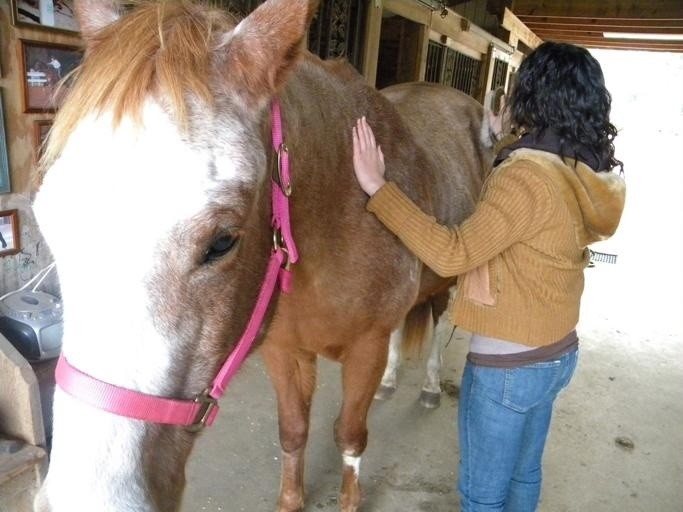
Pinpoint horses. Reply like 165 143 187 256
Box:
30 0 513 512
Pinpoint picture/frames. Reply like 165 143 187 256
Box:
8 0 133 39
0 208 21 257
33 119 55 160
0 85 12 196
16 38 85 113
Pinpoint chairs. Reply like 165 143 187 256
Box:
0 333 50 512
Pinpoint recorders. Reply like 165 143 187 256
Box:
0 288 65 362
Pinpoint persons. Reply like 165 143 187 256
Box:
46 57 62 79
350 39 625 512
